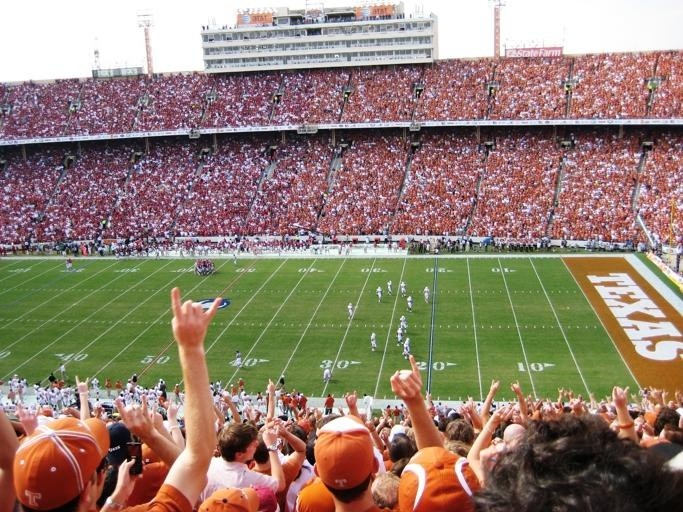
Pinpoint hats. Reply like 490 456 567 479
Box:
314 416 375 490
13 417 129 509
399 446 481 512
197 488 260 512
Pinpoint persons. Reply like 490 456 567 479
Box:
0 50 683 512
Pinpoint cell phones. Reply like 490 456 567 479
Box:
125 441 143 476
